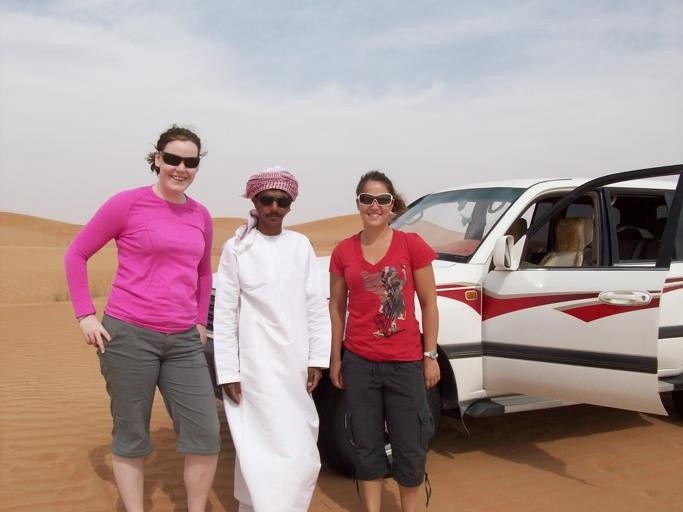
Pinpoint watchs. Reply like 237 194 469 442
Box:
424 351 439 360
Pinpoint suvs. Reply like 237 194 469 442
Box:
205 164 683 476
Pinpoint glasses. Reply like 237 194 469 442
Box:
256 195 291 208
159 151 200 168
356 193 393 207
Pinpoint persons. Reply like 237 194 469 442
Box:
64 124 221 512
213 167 332 512
329 171 441 511
